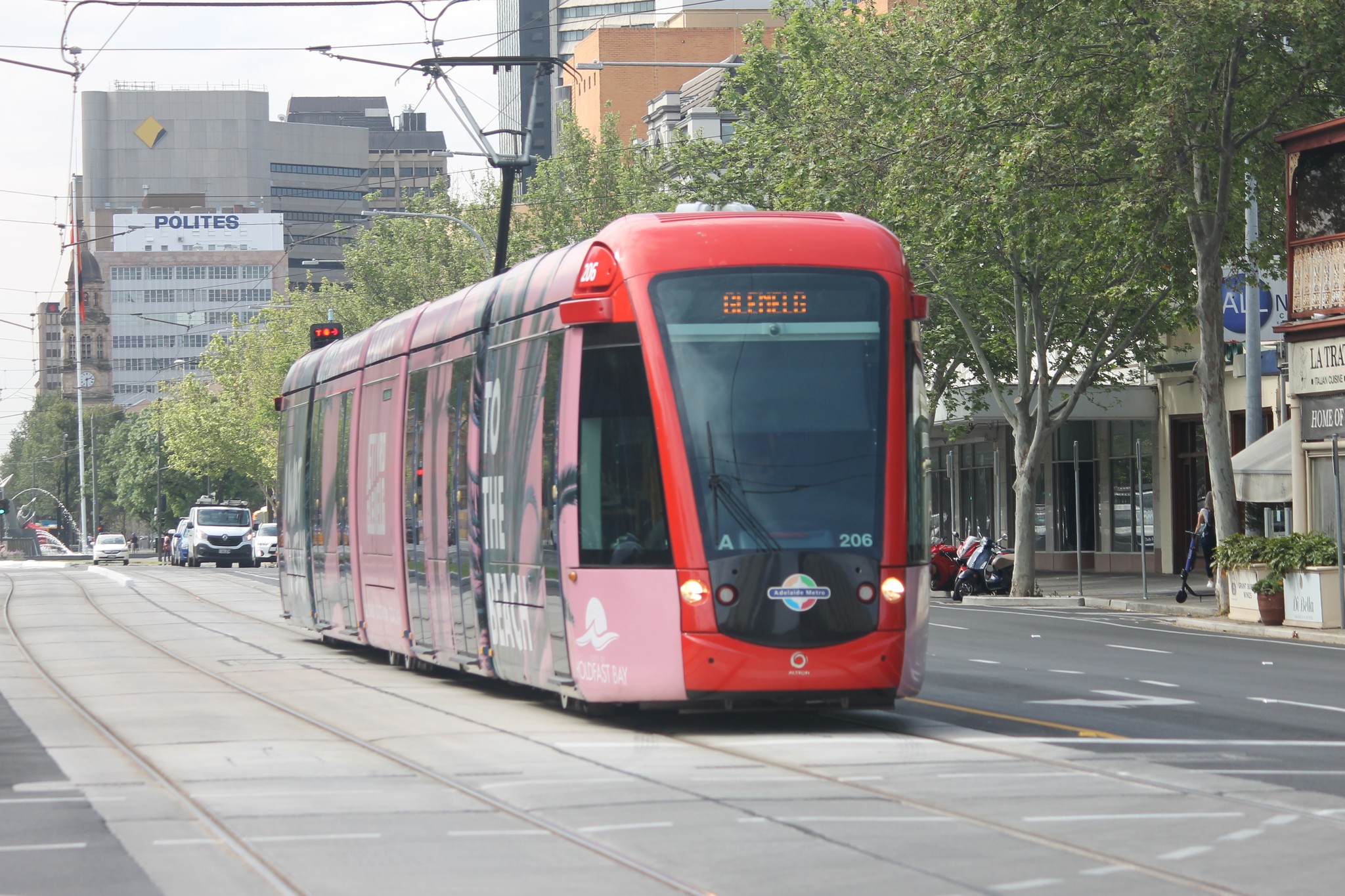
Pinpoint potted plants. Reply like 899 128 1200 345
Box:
1260 532 1345 630
1252 573 1285 626
1207 533 1274 623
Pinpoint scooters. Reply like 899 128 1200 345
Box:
952 531 1015 601
930 525 960 592
954 525 1015 602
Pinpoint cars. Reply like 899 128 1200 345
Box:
174 523 189 566
1035 504 1046 541
90 532 131 565
1099 504 1154 549
167 517 190 565
253 522 278 568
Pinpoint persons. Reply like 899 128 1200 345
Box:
130 533 138 553
163 532 172 563
1195 491 1216 588
87 533 93 548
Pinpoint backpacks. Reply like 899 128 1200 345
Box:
1205 508 1216 536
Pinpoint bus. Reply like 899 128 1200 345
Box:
271 56 929 733
251 506 277 528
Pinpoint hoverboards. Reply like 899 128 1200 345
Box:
1175 530 1215 603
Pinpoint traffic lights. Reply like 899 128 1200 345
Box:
4 498 10 514
0 498 5 514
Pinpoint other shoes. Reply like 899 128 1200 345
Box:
1207 580 1215 588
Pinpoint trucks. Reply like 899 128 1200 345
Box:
187 492 253 568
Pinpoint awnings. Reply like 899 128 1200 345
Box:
1226 416 1292 502
